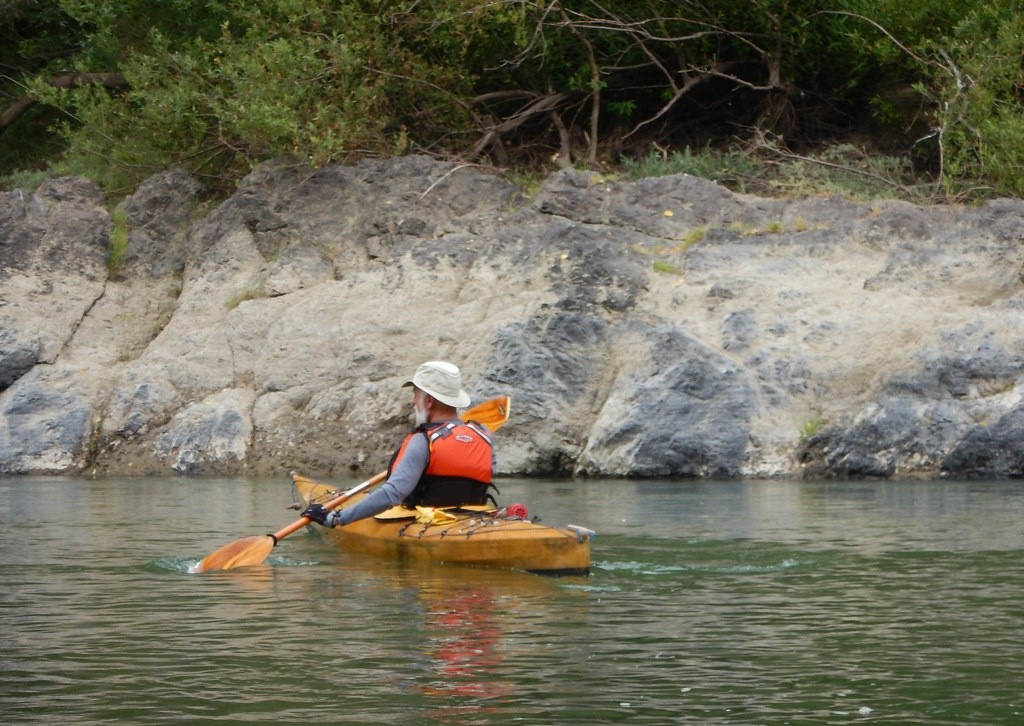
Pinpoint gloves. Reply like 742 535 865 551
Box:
300 502 336 529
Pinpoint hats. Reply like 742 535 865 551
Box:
402 360 472 409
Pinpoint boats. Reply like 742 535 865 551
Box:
286 469 594 578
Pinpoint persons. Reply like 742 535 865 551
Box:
301 361 497 530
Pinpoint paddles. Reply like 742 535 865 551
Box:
189 393 512 573
